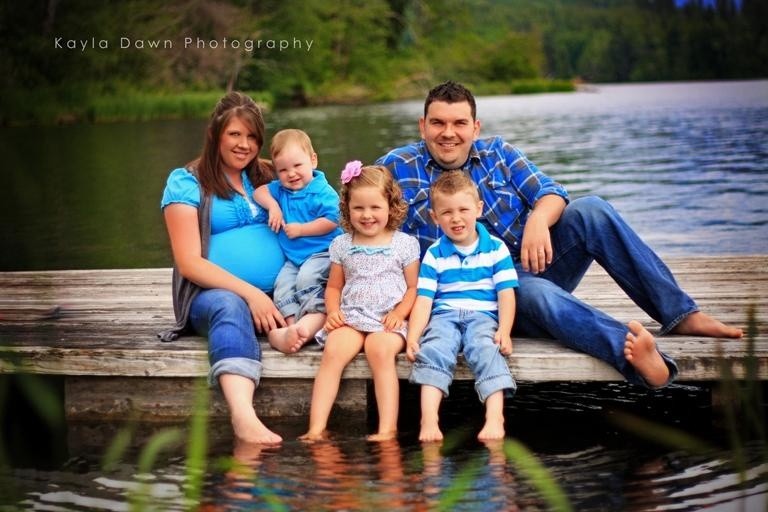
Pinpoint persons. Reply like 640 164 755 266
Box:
299 165 421 441
156 93 327 445
252 128 345 326
405 171 520 441
373 82 744 389
